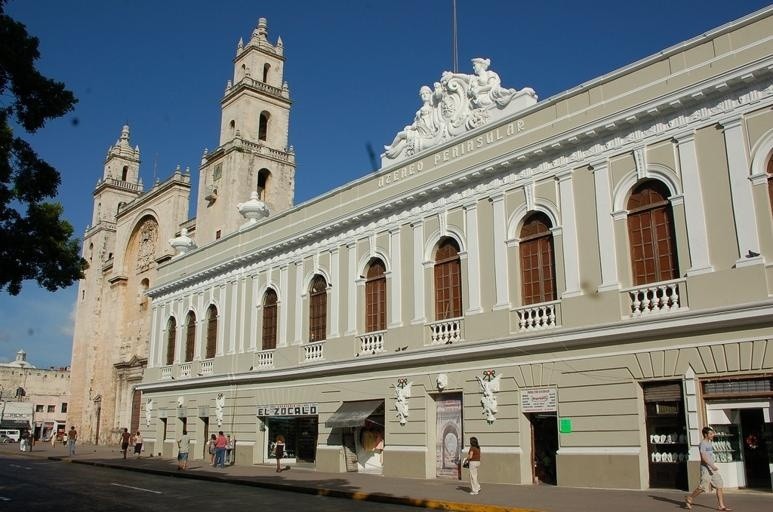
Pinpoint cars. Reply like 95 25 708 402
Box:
0 435 11 444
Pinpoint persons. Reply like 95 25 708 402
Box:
383 85 444 159
206 434 217 466
441 58 515 108
684 427 732 511
43 426 78 456
464 437 481 495
20 429 30 452
119 428 131 460
213 430 228 469
176 430 190 471
271 435 287 473
132 431 142 458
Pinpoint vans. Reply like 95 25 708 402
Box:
0 429 20 443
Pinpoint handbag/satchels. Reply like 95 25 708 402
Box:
463 459 469 468
